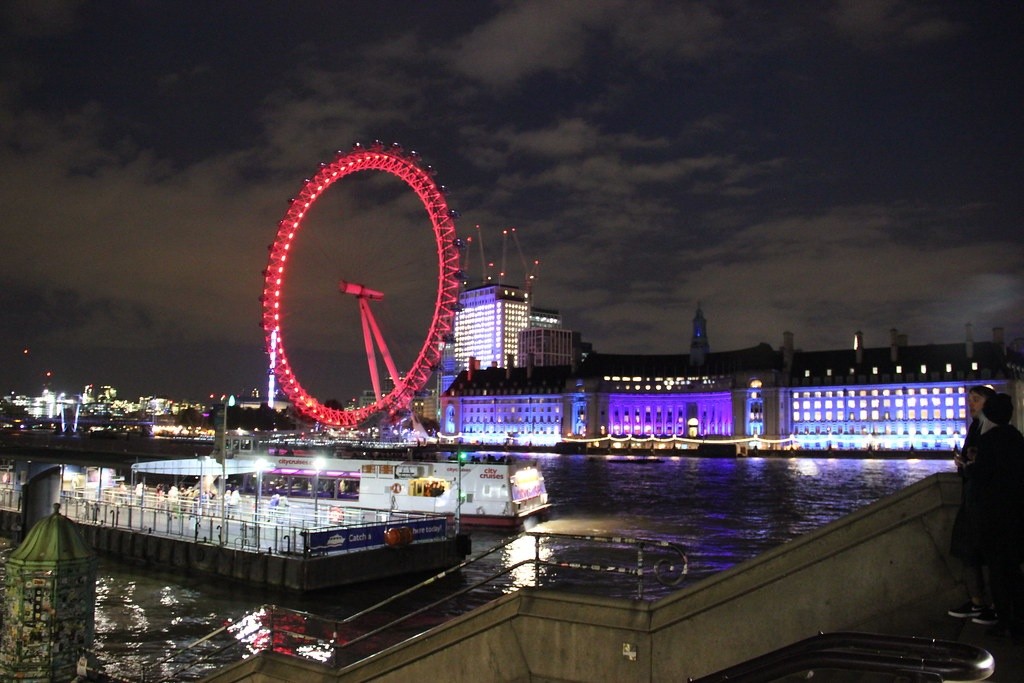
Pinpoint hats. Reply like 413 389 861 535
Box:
981 393 1015 426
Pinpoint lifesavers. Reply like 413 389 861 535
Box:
398 526 413 544
391 482 402 495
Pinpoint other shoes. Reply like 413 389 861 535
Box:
947 595 991 620
971 606 1000 627
984 623 1013 646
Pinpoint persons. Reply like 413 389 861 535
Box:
224 490 241 518
136 482 146 505
471 454 512 465
156 483 213 512
267 494 289 523
119 482 128 503
275 476 360 493
423 482 444 497
